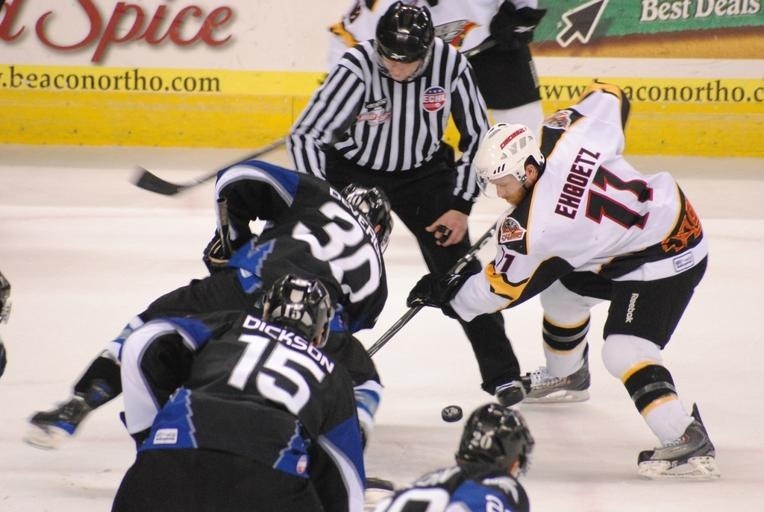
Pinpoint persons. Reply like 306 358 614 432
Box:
28 160 395 437
114 276 370 512
375 402 533 510
328 1 552 152
404 82 721 464
288 2 526 407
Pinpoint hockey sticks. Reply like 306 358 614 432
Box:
129 37 495 196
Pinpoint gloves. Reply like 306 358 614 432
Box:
490 1 547 61
406 271 471 319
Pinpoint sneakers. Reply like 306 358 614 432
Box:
521 346 590 399
495 381 526 407
30 399 89 432
637 402 715 464
365 477 393 490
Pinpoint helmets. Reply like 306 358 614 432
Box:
454 403 535 480
340 182 395 254
374 1 435 83
260 274 333 349
473 122 545 199
